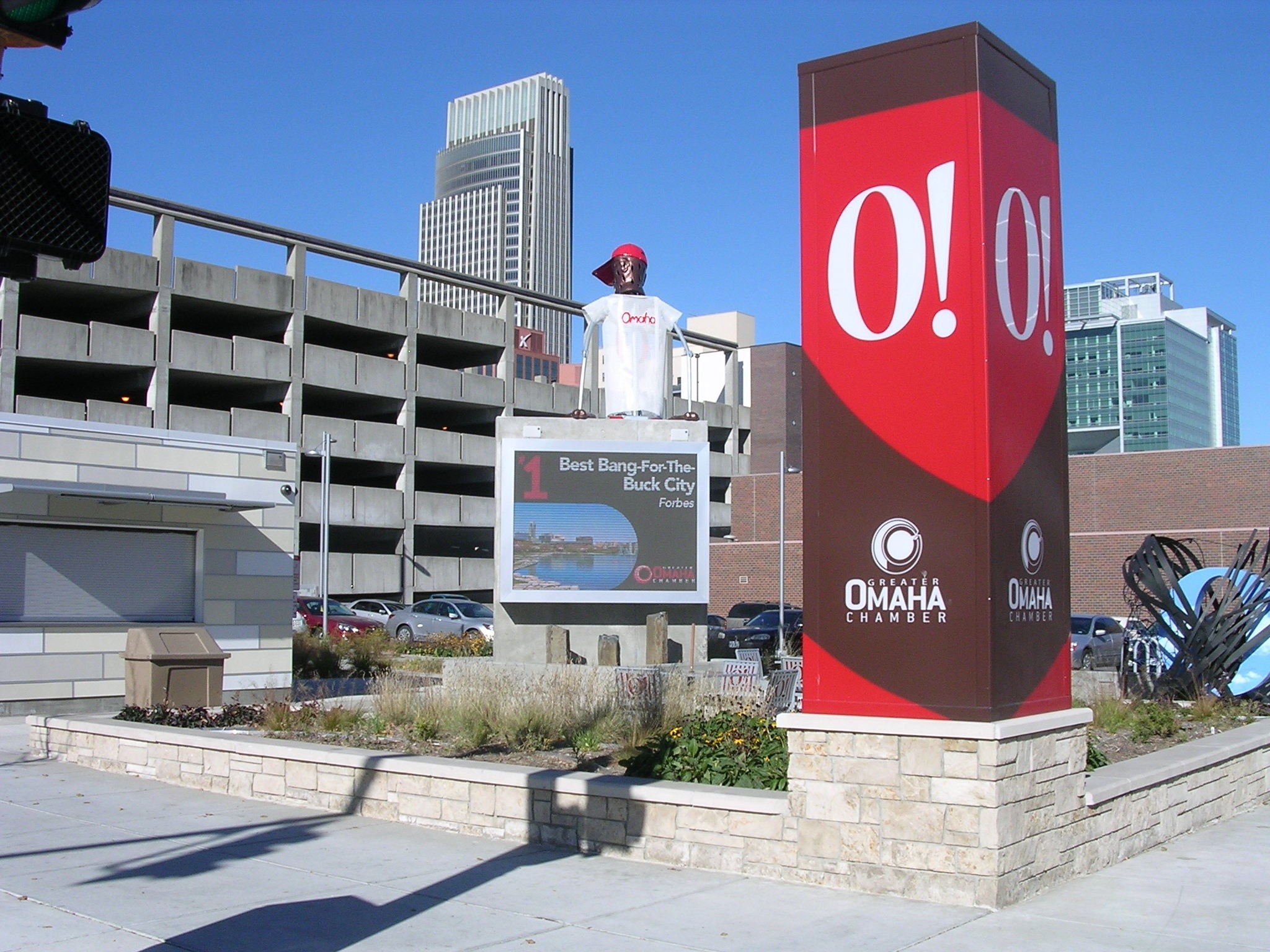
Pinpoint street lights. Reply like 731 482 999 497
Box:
777 451 800 653
305 433 337 642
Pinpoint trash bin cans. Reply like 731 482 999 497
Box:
119 627 231 709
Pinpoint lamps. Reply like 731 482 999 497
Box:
442 426 448 430
121 397 130 402
387 352 394 358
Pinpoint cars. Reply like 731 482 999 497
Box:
1111 616 1150 643
384 599 494 646
343 598 408 626
295 596 391 649
708 614 727 657
1070 613 1132 671
715 608 806 663
428 593 470 603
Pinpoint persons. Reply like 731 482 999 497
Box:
572 244 699 421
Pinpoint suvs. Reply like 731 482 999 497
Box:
724 600 803 631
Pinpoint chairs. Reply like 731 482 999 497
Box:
737 668 802 720
611 667 679 727
311 604 320 615
438 605 449 617
734 646 768 683
463 607 475 616
779 656 803 710
700 657 762 717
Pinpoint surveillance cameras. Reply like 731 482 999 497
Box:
281 485 291 495
